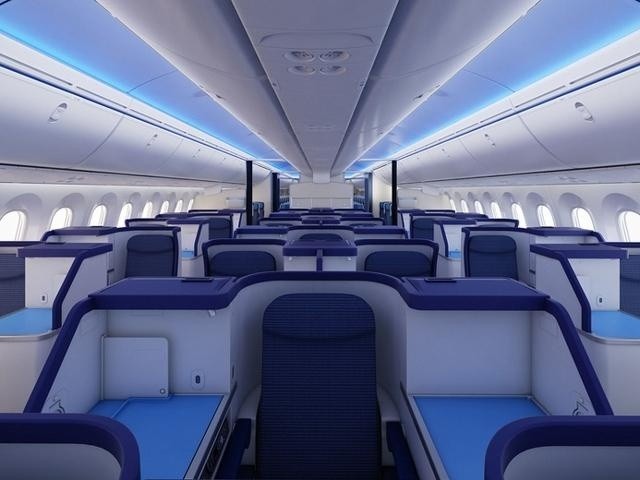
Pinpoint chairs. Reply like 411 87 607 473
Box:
234 225 407 242
462 228 606 286
0 276 238 479
0 238 114 337
252 202 265 225
410 213 489 242
380 201 417 228
271 211 372 217
380 277 640 480
229 270 398 480
259 217 385 226
278 209 365 212
397 209 455 237
188 209 247 232
155 213 233 238
201 240 286 278
355 237 440 281
530 242 640 338
432 219 519 277
125 219 209 277
41 226 181 285
